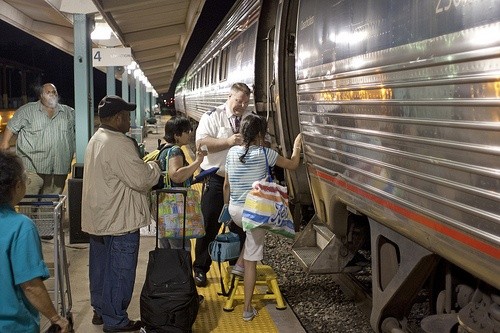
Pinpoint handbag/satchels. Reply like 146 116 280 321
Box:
210 224 240 263
151 187 206 239
242 177 295 243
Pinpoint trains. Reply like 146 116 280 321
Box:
173 0 500 291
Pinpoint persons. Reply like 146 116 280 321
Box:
193 83 260 288
0 83 75 240
223 113 301 321
150 116 208 307
0 149 69 333
81 96 161 333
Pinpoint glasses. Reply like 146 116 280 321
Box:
21 177 31 185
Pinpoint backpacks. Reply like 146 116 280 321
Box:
142 144 172 221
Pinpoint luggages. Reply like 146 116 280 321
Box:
140 189 199 333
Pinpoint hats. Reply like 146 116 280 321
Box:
98 95 136 118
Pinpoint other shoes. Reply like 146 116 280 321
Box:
242 305 258 322
103 319 142 333
93 309 104 325
232 264 245 277
194 268 208 287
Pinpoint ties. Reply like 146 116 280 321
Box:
235 116 240 133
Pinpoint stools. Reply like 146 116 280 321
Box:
218 264 287 313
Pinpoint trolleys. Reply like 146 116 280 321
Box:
15 194 74 333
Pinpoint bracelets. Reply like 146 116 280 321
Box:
51 316 61 323
293 147 301 150
